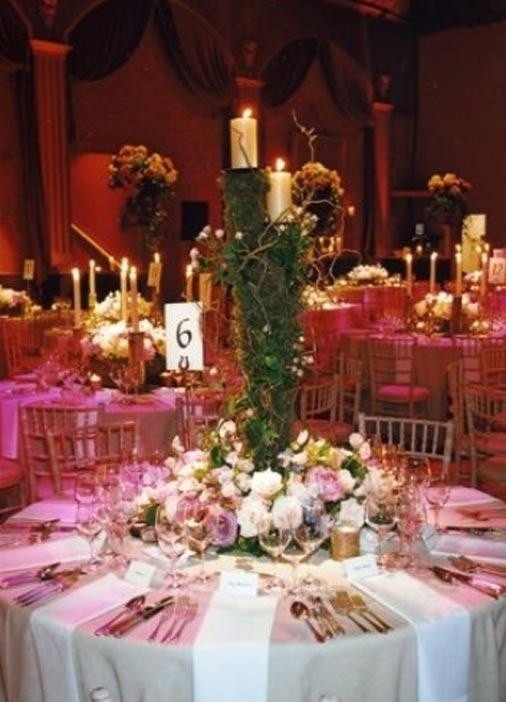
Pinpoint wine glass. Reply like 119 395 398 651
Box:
73 446 218 595
32 346 146 408
256 432 451 595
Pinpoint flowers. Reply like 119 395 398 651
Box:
107 143 180 266
427 172 472 212
76 416 409 558
293 161 345 236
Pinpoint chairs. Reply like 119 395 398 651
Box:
0 287 506 505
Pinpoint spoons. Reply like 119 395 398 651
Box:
94 595 146 635
290 601 324 644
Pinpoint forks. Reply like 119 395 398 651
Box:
329 588 395 636
149 596 199 646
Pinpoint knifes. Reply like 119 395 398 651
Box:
14 569 79 607
307 595 343 639
107 592 175 636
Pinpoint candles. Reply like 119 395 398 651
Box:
404 241 492 334
72 247 208 326
225 106 294 234
